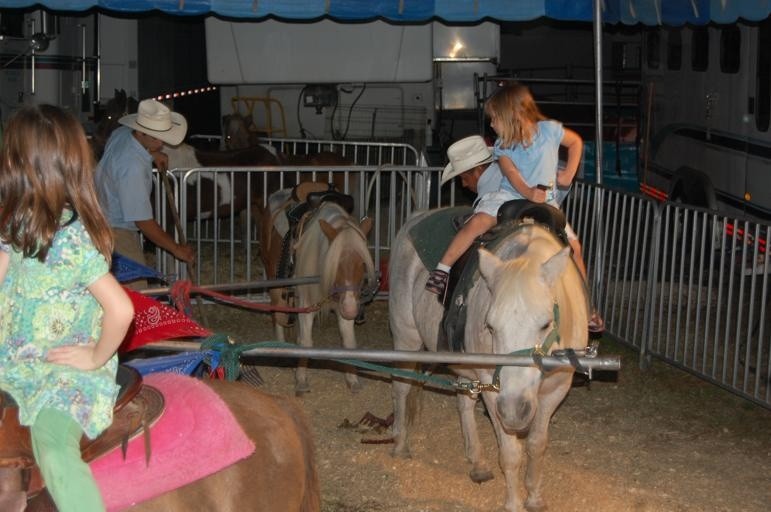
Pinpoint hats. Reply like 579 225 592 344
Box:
441 135 494 187
117 99 188 146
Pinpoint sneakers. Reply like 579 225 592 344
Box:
588 307 605 333
425 269 448 295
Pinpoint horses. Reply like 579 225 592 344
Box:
387 207 592 512
92 89 292 227
259 187 375 398
221 112 358 256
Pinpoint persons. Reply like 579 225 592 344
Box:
424 79 605 333
91 98 194 295
438 135 496 194
0 101 135 512
